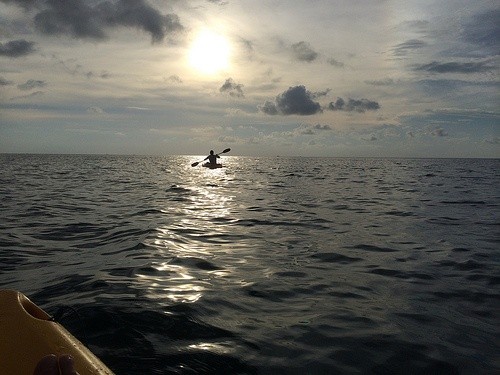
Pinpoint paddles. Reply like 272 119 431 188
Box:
191 148 231 167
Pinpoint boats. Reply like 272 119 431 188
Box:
202 162 222 168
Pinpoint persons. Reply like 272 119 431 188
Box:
204 150 220 164
